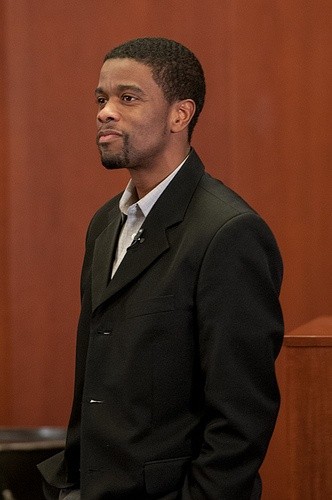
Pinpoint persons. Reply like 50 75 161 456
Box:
36 39 285 500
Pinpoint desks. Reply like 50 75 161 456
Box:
259 316 332 500
